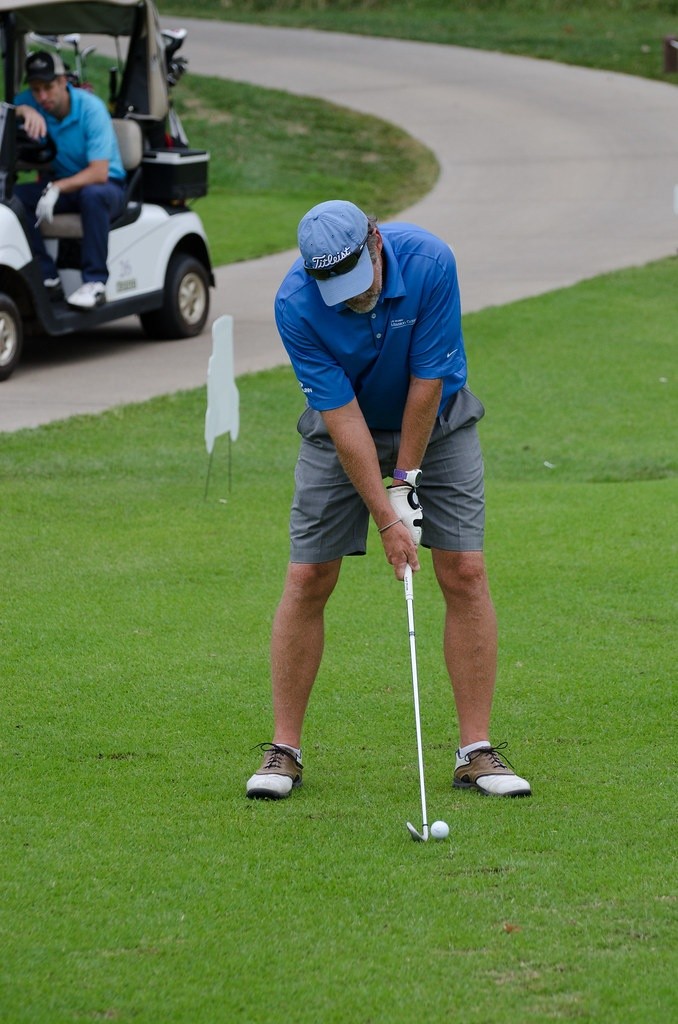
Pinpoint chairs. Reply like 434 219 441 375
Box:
35 117 144 239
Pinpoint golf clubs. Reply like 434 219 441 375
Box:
404 565 429 842
64 30 96 86
166 55 188 88
430 821 449 838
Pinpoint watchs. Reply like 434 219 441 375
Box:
393 468 422 487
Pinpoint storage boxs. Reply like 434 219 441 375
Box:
141 147 211 201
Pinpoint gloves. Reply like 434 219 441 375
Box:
35 186 60 225
386 484 423 547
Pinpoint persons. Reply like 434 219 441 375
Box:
244 201 530 800
15 49 128 310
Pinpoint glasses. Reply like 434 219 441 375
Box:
302 227 375 279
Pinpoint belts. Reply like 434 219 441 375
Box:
436 392 458 426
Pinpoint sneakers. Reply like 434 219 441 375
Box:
246 742 303 799
67 279 105 308
452 742 532 798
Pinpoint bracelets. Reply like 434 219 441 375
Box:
378 519 401 532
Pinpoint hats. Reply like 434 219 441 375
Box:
24 50 65 84
297 200 376 307
